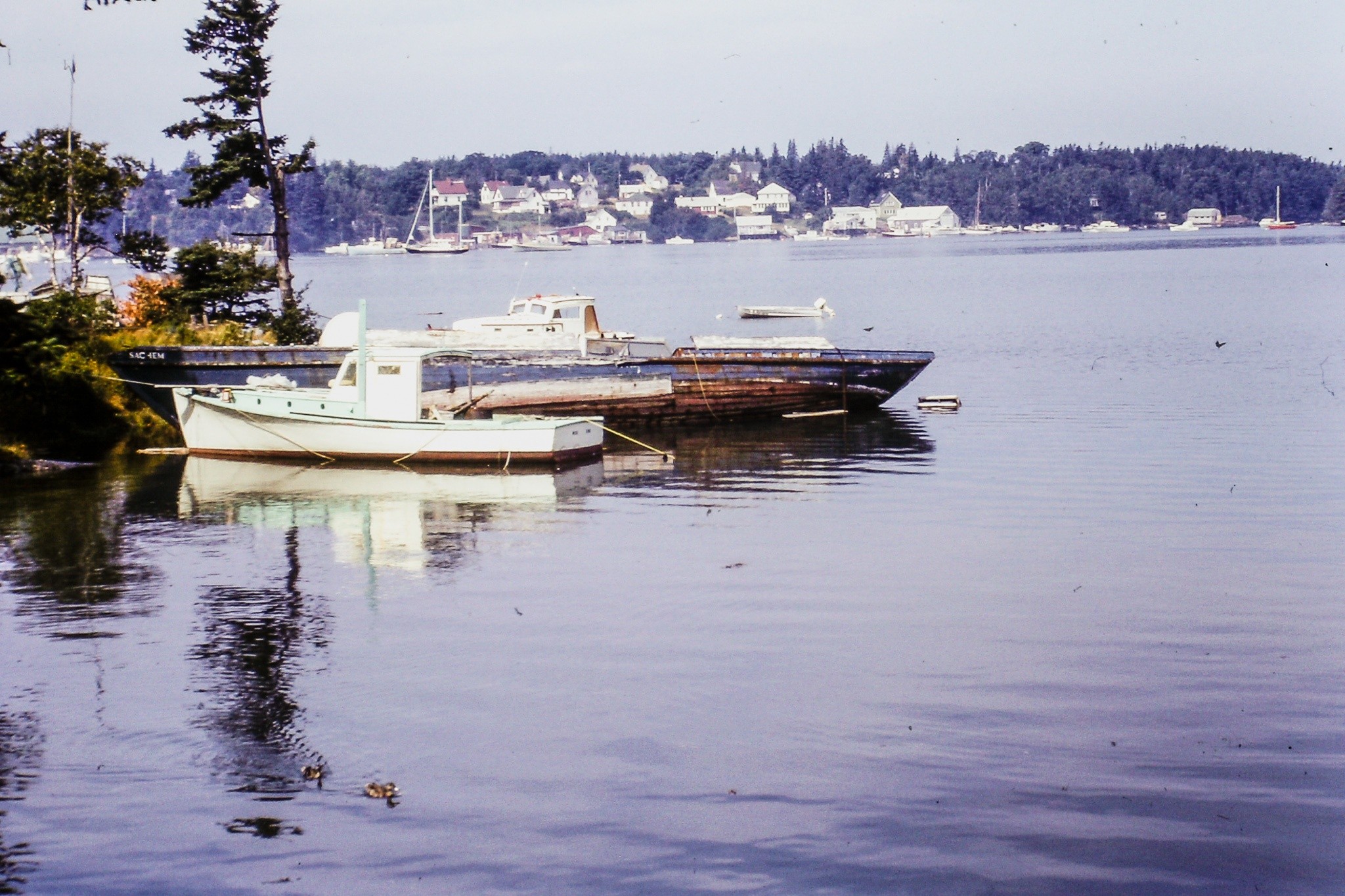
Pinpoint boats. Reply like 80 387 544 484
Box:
1170 221 1199 232
736 297 835 318
1024 222 1061 232
665 236 694 245
491 237 519 248
451 293 633 355
511 235 572 253
1259 218 1275 227
1080 221 1130 233
794 230 850 240
562 234 611 245
108 311 934 432
324 237 407 256
882 226 1017 236
173 298 605 468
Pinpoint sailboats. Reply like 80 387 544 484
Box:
961 181 997 234
402 169 470 254
1268 186 1297 229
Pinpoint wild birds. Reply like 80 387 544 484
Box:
1215 340 1227 349
863 327 874 332
515 608 522 616
706 507 721 517
1091 356 1108 370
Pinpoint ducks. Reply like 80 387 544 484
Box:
301 764 324 780
386 792 402 808
364 782 400 798
